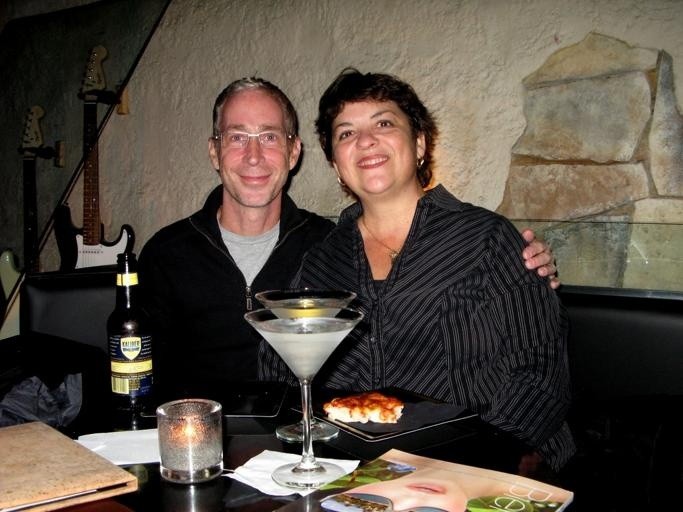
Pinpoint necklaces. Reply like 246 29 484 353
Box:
362 216 400 265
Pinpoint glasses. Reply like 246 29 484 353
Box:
212 131 293 147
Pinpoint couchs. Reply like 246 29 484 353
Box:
16 257 682 512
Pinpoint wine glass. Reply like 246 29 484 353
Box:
249 286 360 442
242 305 364 495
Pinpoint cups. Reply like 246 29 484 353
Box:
157 399 229 485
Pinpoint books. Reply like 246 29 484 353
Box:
271 447 575 512
0 422 139 511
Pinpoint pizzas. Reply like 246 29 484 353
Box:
323 390 405 424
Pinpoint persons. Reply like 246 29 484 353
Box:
134 75 561 386
320 448 573 512
511 451 541 476
250 67 578 478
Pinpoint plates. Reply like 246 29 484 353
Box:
288 386 481 445
139 379 290 418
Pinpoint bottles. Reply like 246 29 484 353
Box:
112 412 154 498
105 254 153 413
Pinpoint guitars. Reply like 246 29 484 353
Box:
1 107 51 339
53 46 135 268
